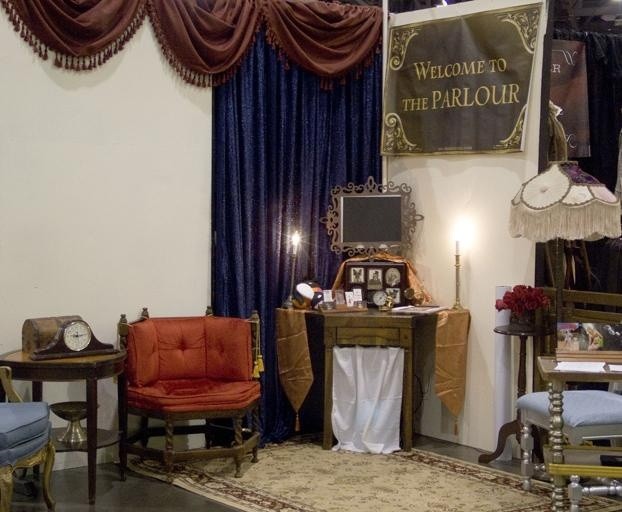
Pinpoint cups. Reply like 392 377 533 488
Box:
335 290 344 305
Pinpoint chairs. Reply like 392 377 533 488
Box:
0 367 55 511
113 303 263 485
475 376 622 512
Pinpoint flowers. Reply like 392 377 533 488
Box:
491 282 552 312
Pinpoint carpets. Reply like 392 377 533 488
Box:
113 447 622 511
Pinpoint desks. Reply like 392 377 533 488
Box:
533 353 622 511
0 348 129 505
479 325 554 465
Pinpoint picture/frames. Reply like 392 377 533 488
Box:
349 267 383 292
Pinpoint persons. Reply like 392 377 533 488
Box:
369 271 381 285
587 328 603 350
561 331 574 348
606 325 620 341
